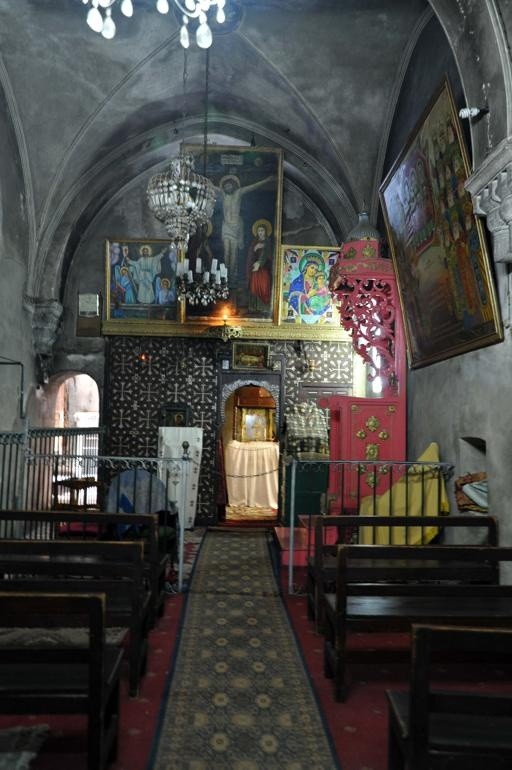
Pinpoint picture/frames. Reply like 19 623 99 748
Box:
178 142 284 332
104 233 184 325
376 69 505 369
277 245 347 329
231 341 271 373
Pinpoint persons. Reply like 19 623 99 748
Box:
175 415 186 426
111 173 338 323
395 117 494 331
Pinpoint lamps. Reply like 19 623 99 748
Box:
150 26 217 243
75 1 226 54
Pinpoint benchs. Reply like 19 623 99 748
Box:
307 513 511 770
0 508 178 768
